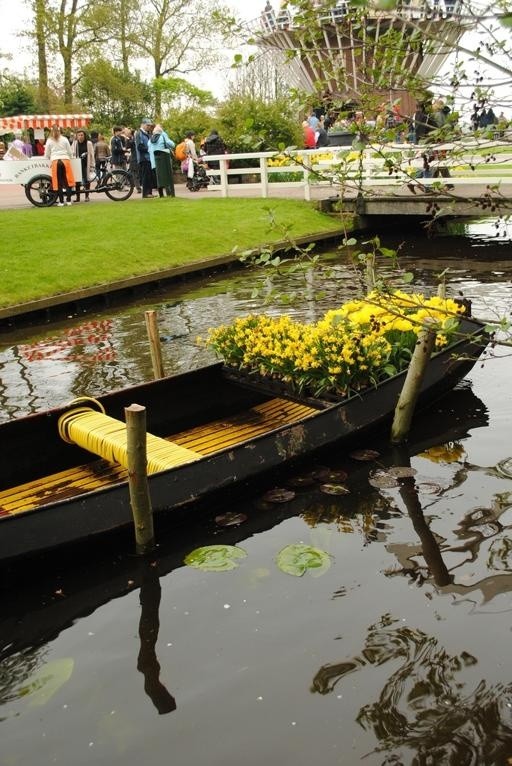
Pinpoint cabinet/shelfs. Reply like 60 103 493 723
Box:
0 155 91 186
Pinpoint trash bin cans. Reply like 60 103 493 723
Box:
154 150 175 198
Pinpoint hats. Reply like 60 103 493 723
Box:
142 118 152 126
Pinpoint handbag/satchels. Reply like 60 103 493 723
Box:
88 171 99 182
181 158 189 171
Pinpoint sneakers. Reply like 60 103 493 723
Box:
407 184 455 194
57 202 71 206
122 185 130 191
137 188 158 198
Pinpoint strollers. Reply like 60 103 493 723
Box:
185 154 215 192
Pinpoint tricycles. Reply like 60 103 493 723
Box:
0 113 136 207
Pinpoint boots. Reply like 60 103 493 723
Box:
84 183 90 202
73 185 80 202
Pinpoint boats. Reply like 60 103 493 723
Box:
2 304 493 564
0 379 486 665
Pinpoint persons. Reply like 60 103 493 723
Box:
1 117 231 207
299 100 506 149
263 0 276 25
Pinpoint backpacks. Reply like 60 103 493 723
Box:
176 139 191 161
